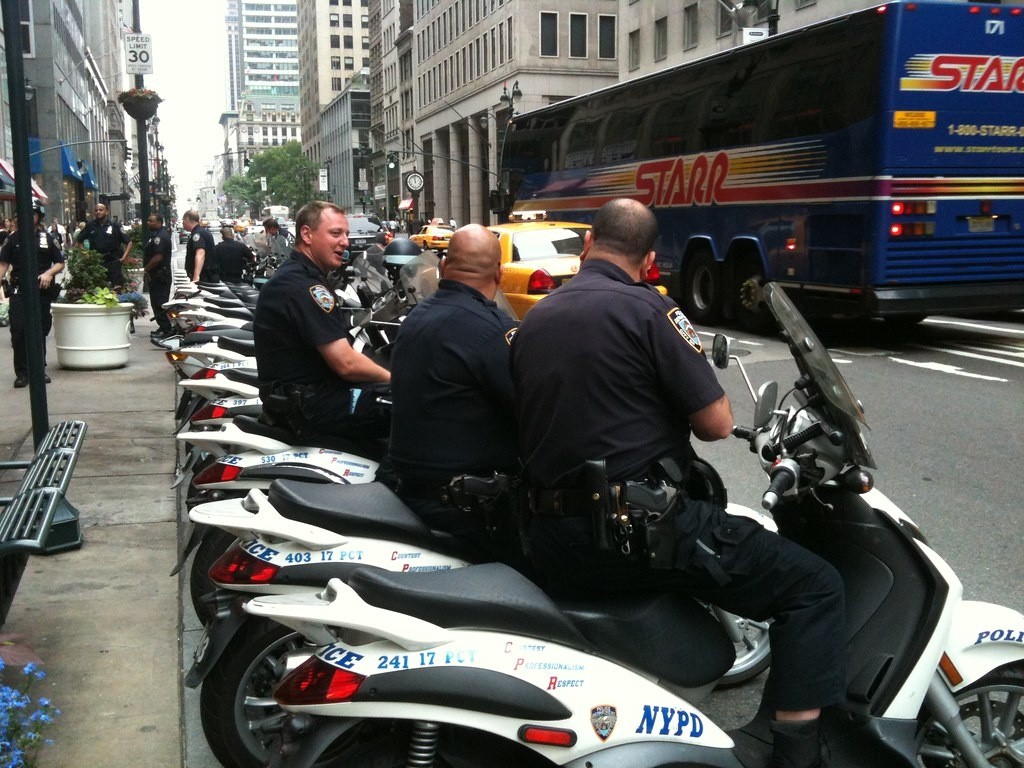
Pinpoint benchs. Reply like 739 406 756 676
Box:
0 420 85 630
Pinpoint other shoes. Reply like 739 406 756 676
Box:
13 375 26 388
150 328 168 338
769 720 831 767
46 374 51 382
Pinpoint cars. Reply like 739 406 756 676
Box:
343 212 390 270
484 220 668 324
406 223 457 259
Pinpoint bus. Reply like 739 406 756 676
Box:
483 0 1024 346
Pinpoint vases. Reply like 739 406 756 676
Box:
122 99 158 120
49 302 135 370
120 268 145 297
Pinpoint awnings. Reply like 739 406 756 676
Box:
62 143 98 191
0 159 48 199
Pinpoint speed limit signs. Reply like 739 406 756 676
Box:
124 33 154 75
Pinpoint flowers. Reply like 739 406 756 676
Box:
118 88 164 104
117 227 145 270
48 247 148 318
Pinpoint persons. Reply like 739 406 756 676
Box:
253 201 393 439
508 197 849 768
382 218 407 234
388 224 528 542
263 218 294 238
76 203 135 334
182 210 220 283
142 212 173 336
215 227 254 284
0 197 90 388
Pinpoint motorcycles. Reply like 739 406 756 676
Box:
149 209 1024 768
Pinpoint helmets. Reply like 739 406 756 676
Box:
382 238 421 265
31 195 46 217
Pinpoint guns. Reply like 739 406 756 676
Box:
621 477 681 571
450 470 513 549
